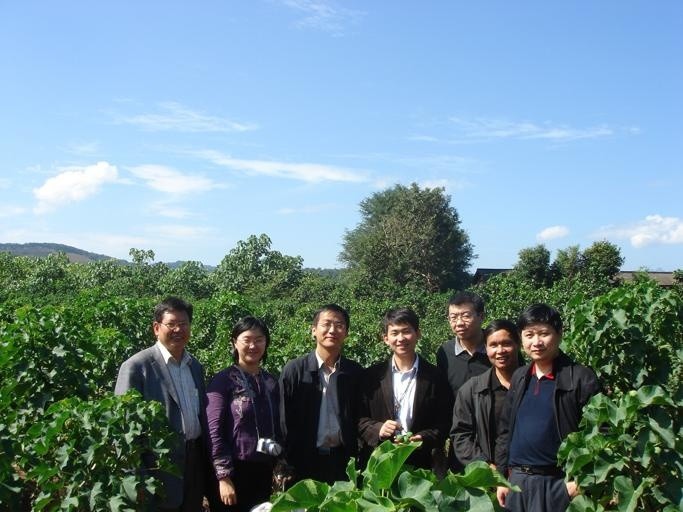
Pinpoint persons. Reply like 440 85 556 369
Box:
449 319 526 479
435 290 526 419
113 296 207 512
356 307 454 472
279 303 365 492
205 316 283 512
495 303 601 512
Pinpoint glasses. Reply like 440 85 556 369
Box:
310 321 345 331
153 319 190 331
229 338 266 348
444 312 480 324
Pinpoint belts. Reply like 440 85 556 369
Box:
316 446 343 455
509 463 562 474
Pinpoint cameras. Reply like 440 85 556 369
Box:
256 438 282 456
